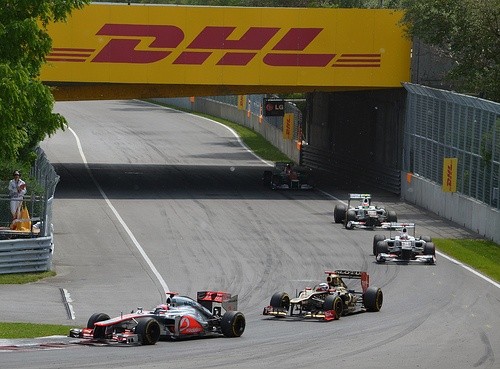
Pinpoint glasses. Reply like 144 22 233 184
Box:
13 174 21 176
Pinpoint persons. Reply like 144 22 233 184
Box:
154 304 170 315
319 283 330 293
400 234 409 241
362 203 369 210
7 170 27 220
286 168 292 174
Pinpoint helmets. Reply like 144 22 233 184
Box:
400 234 408 240
319 283 329 291
156 304 168 312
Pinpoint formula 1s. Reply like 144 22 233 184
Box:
68 291 246 346
261 270 383 321
333 193 397 230
266 161 323 191
370 221 436 266
